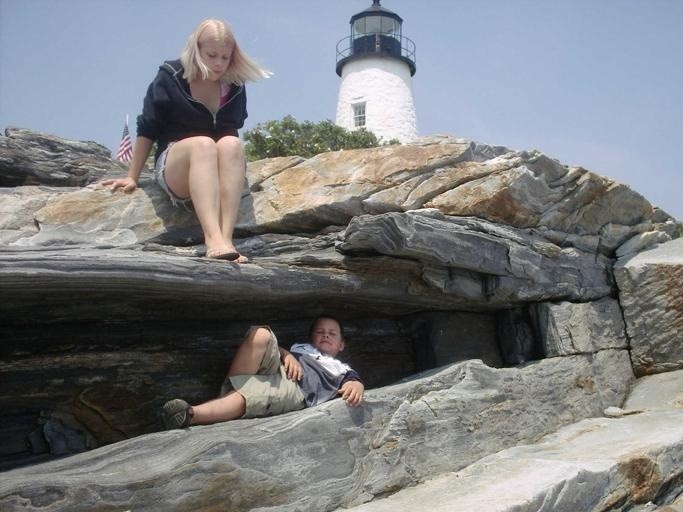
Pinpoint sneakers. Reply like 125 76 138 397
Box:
233 255 249 264
204 249 240 261
159 398 194 429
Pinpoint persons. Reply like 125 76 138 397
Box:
154 313 365 432
100 17 274 265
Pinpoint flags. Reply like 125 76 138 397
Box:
115 117 133 163
